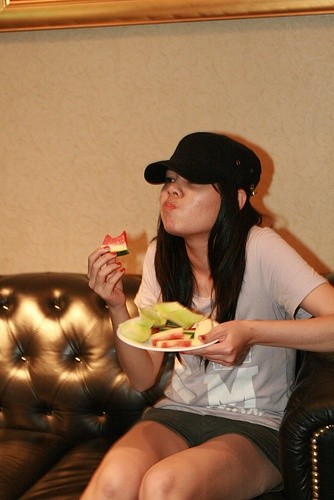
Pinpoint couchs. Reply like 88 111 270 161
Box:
0 273 334 500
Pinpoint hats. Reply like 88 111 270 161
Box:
143 131 262 197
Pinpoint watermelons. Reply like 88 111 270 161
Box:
147 326 191 349
100 231 129 256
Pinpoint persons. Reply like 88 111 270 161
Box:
74 131 334 500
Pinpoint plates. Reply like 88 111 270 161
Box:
116 317 222 351
